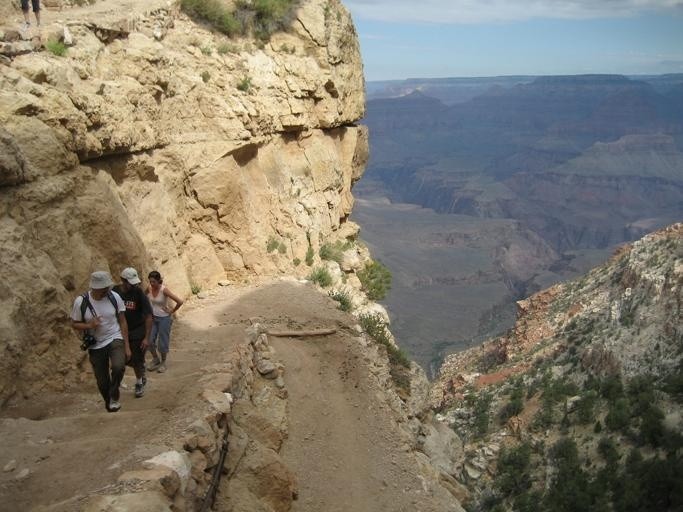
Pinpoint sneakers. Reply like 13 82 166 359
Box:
146 359 167 373
108 396 121 411
133 373 147 397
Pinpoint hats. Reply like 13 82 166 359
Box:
120 266 142 286
88 270 115 290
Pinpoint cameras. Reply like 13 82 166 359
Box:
80 334 97 351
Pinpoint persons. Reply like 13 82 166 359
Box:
143 271 183 372
112 267 154 398
67 271 132 412
